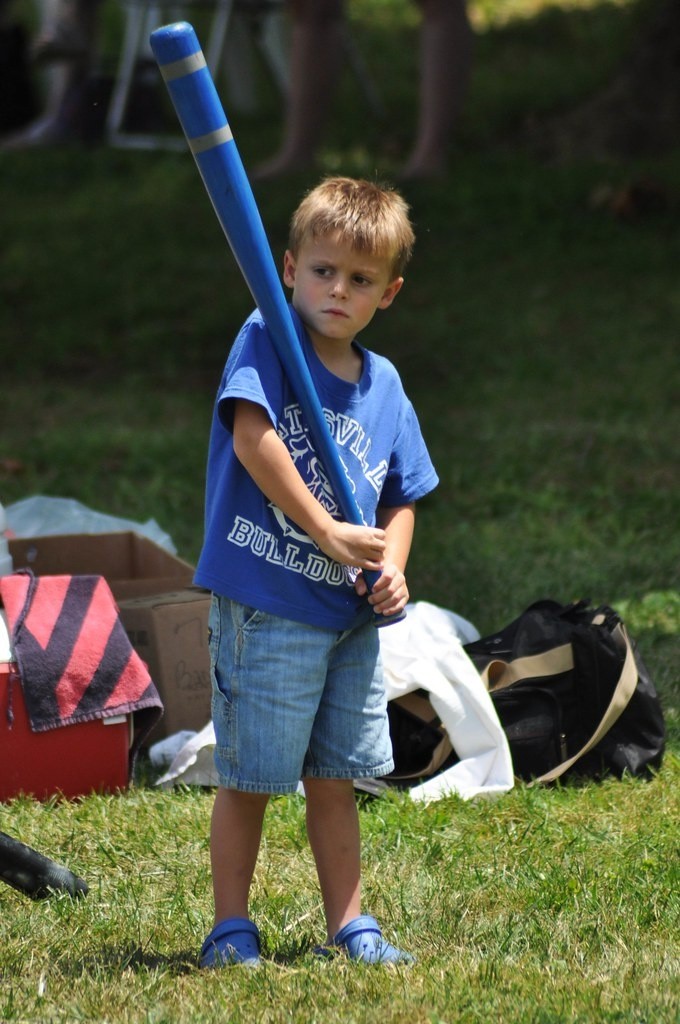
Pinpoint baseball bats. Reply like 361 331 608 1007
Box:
150 20 407 630
0 831 90 905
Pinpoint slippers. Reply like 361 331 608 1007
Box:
319 917 417 968
199 918 261 971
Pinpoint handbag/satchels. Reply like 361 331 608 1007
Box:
376 597 664 788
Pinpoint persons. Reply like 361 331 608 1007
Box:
192 172 442 970
254 0 470 180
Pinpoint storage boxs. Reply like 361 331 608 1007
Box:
0 530 210 801
